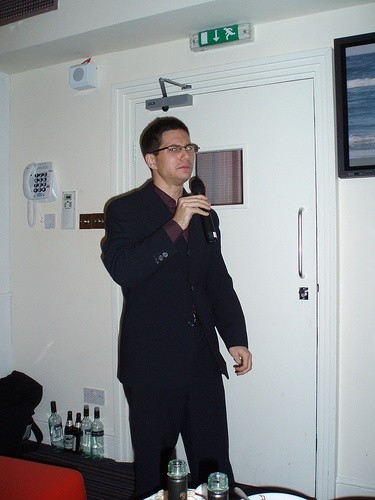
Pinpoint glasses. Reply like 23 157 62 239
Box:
155 143 200 155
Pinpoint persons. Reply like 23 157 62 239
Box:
99 117 253 500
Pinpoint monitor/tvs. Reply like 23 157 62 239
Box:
333 31 375 179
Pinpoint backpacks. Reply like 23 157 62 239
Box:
0 371 45 456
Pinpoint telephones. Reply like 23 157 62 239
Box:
23 162 57 202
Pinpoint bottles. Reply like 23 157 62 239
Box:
62 411 75 449
166 459 189 500
91 407 104 455
48 401 64 448
81 405 92 454
208 472 229 500
72 412 82 451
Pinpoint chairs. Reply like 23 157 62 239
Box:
0 455 88 500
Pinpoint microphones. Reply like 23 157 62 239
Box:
188 175 217 245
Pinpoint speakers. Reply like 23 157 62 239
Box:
68 62 98 90
